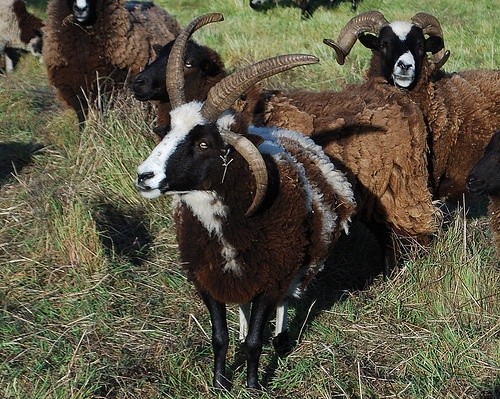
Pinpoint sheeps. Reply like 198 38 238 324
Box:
0 0 44 77
322 10 500 271
40 0 182 136
129 37 438 263
133 11 357 396
